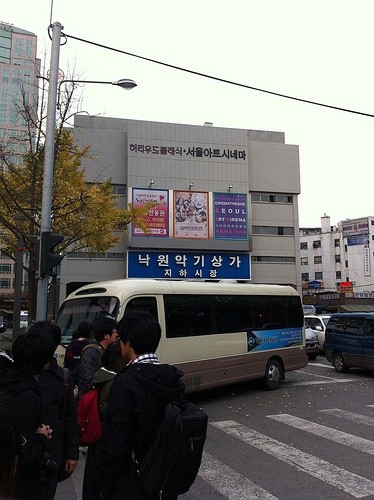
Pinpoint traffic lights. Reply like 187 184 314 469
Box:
39 232 65 275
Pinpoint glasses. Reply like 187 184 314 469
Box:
111 332 118 333
116 335 121 342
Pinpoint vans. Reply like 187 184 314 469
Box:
323 310 374 374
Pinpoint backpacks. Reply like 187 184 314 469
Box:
77 388 102 444
147 401 209 494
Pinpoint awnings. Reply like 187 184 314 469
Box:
339 305 374 312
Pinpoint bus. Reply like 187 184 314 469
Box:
50 276 309 400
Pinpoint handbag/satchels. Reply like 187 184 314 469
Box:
44 439 74 483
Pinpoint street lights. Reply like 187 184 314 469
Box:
34 77 138 324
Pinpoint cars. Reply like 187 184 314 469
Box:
0 313 7 333
19 310 28 329
302 304 333 362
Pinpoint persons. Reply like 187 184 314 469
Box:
0 321 80 500
63 310 184 500
